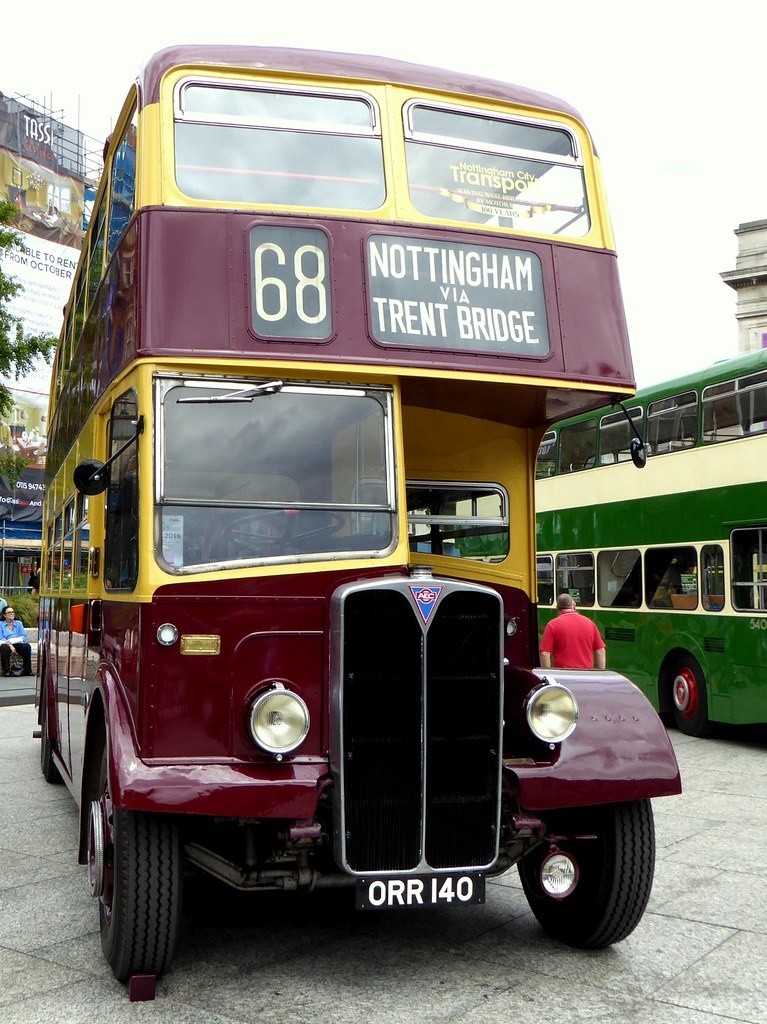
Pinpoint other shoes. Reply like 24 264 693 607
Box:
28 672 35 676
4 672 9 677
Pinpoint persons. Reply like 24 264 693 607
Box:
31 568 41 595
539 593 607 669
0 606 33 676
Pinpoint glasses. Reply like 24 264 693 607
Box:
5 611 15 614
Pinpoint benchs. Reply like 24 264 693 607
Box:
547 439 717 477
10 628 38 675
671 593 727 611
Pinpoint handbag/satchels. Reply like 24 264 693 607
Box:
11 663 24 676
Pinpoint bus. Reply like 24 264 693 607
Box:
33 43 693 985
452 348 767 733
